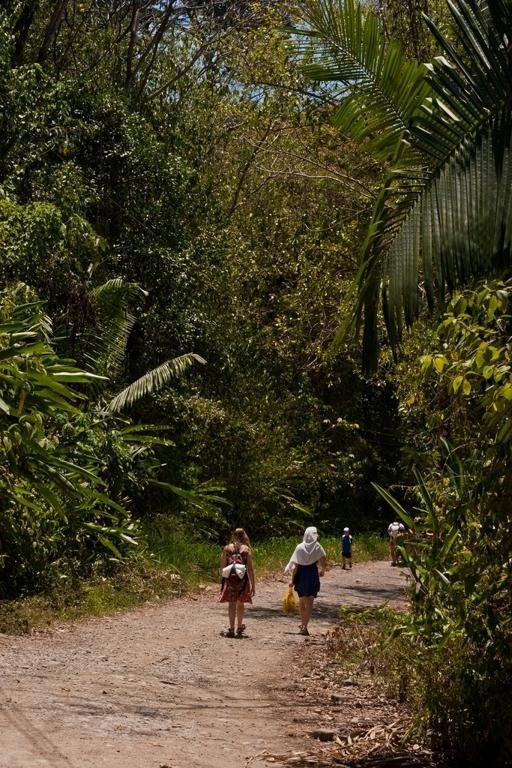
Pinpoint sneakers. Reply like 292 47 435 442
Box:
300 628 310 635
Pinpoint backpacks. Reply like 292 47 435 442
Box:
391 522 400 536
229 544 246 585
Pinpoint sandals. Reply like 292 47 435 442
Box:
236 624 245 634
229 628 235 635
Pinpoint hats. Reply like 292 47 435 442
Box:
343 527 349 532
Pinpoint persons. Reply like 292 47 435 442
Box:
217 526 256 639
283 525 327 635
395 526 408 566
387 516 404 566
340 527 354 569
